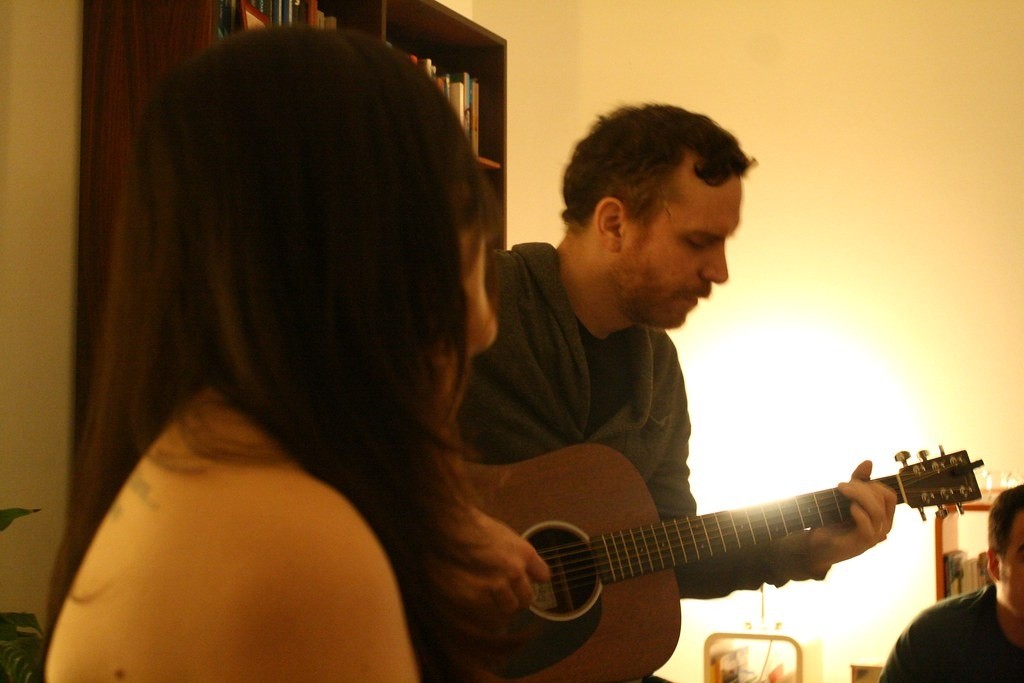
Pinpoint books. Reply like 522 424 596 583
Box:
944 550 988 597
214 0 480 157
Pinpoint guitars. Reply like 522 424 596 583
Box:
416 439 986 683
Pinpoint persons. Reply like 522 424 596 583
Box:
424 105 897 683
42 22 498 682
878 485 1024 683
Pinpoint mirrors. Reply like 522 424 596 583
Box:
703 632 804 683
933 504 994 602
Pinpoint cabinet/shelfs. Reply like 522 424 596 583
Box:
74 0 510 565
